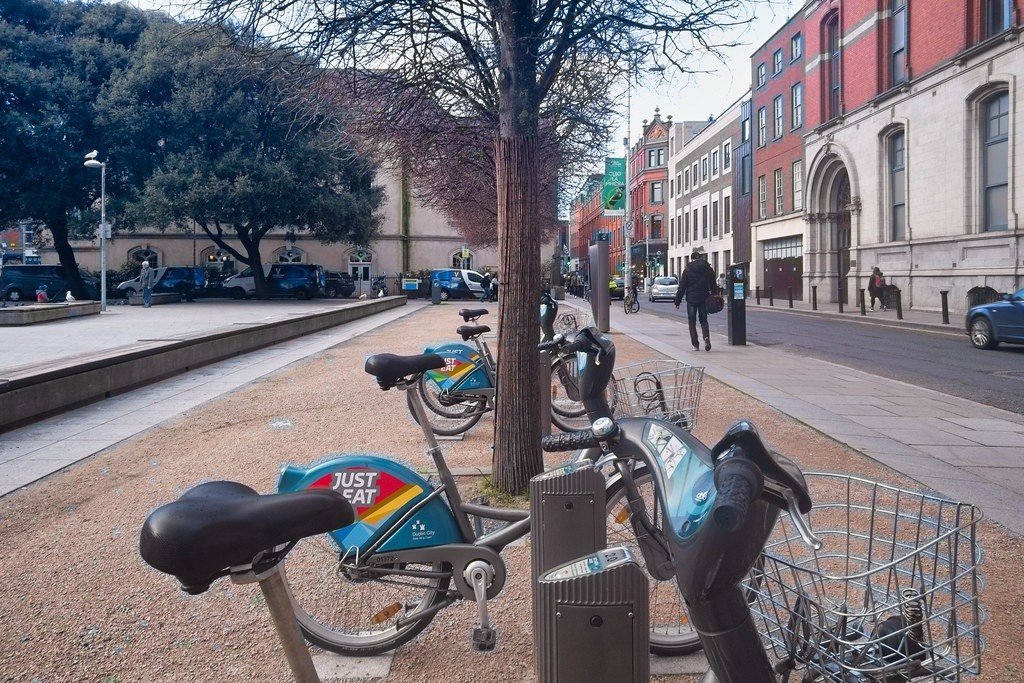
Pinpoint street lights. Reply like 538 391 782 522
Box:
83 160 107 311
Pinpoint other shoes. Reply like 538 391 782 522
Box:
884 305 887 311
705 336 711 351
480 298 484 302
488 300 493 302
692 346 699 351
869 308 874 311
143 304 151 308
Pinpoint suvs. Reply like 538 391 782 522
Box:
431 268 498 302
117 265 203 300
0 262 102 303
219 261 325 299
323 270 357 298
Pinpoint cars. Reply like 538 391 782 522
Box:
647 276 681 303
609 277 625 300
964 287 1024 350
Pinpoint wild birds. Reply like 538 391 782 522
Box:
377 289 384 299
65 291 76 303
358 294 368 301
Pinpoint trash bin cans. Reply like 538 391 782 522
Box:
967 286 999 309
882 285 900 309
432 279 442 304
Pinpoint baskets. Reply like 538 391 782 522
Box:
556 309 592 337
741 473 986 683
604 360 705 431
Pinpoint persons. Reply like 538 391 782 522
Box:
561 271 585 298
175 276 195 303
480 272 499 302
867 267 887 312
139 260 154 308
608 273 725 304
36 285 56 303
674 252 716 351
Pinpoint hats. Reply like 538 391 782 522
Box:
39 283 48 289
691 252 701 259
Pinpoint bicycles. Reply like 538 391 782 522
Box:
139 415 986 683
406 291 619 436
264 325 766 659
623 284 640 314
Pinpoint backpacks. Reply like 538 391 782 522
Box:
481 278 485 288
875 275 883 287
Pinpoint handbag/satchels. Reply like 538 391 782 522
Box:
705 294 724 314
